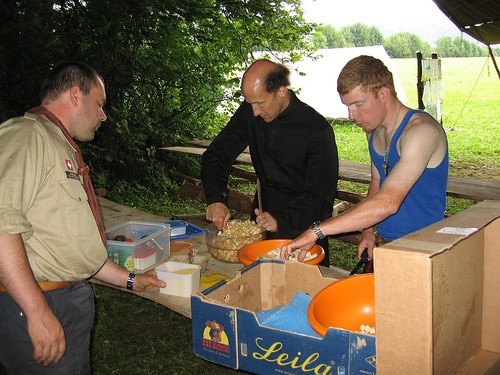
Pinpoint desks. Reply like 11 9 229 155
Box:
159 146 372 185
92 183 349 320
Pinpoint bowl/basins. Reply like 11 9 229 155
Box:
307 270 377 343
238 239 325 274
205 220 267 264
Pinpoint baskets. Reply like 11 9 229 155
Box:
205 219 267 264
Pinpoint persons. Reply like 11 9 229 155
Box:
201 60 339 267
0 63 167 375
281 55 449 272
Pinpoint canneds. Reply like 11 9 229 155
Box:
106 245 135 272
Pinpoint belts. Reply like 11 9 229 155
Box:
0 279 87 294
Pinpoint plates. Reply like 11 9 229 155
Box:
134 219 205 244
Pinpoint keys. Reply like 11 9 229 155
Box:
382 162 388 176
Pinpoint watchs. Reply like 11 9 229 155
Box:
127 272 136 290
310 221 325 240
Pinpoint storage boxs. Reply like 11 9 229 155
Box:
104 221 172 274
373 199 500 375
192 260 376 375
155 261 201 297
